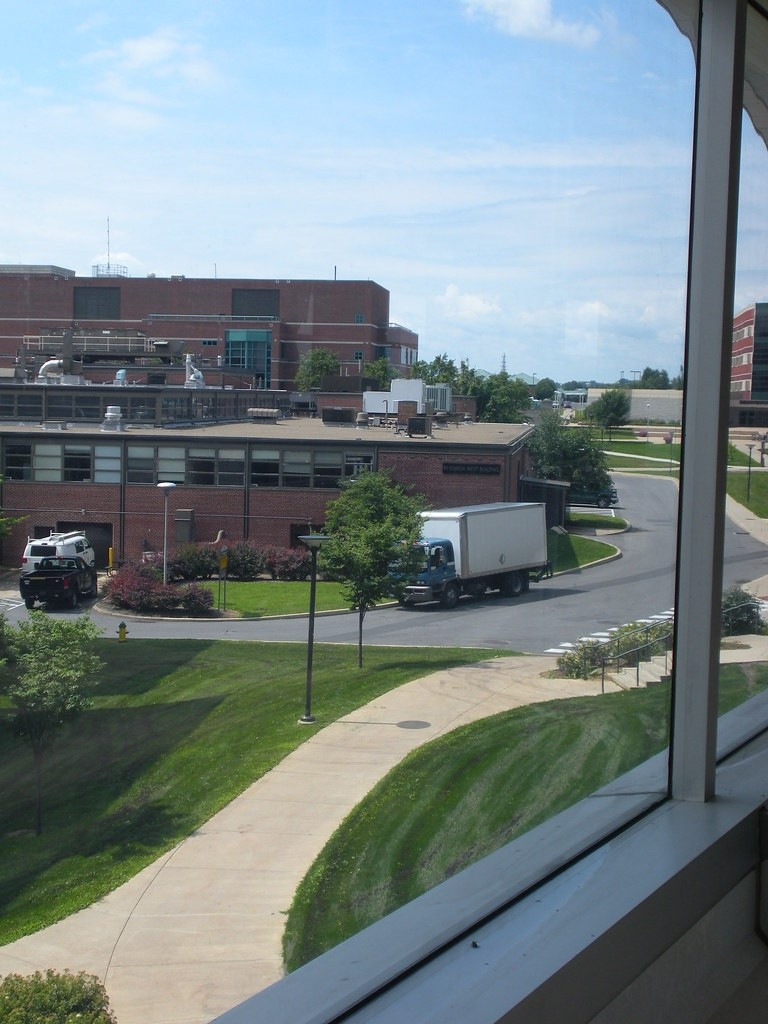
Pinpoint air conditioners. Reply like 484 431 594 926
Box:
362 378 453 414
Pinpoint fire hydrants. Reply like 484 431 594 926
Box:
116 621 129 642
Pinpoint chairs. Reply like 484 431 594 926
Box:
66 561 75 569
431 548 440 566
44 562 53 568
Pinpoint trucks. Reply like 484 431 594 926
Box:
387 501 553 610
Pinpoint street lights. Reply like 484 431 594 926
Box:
646 404 651 442
298 535 331 722
157 482 177 586
746 444 756 502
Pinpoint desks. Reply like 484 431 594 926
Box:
112 559 127 570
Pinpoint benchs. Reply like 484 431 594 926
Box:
105 566 113 576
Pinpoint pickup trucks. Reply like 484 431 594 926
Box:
19 556 97 610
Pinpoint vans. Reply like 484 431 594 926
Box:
21 530 95 576
